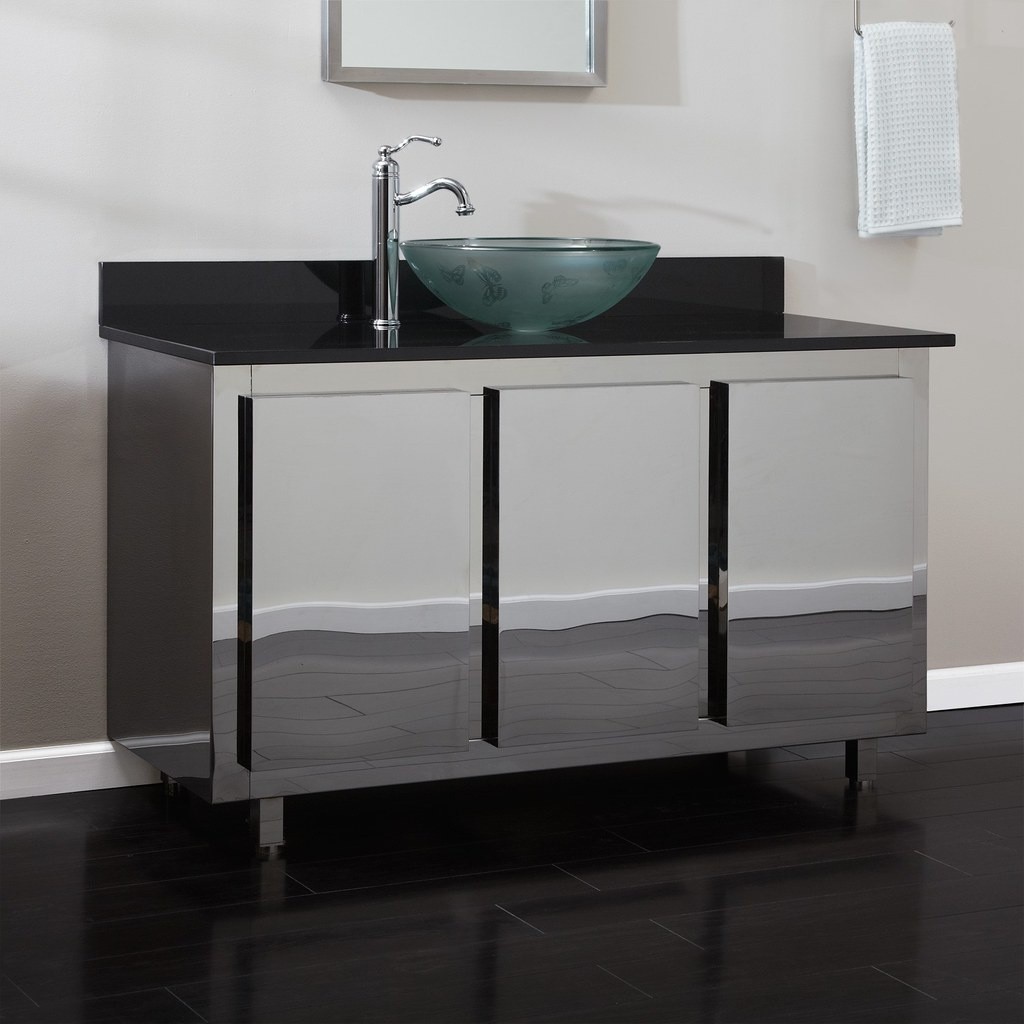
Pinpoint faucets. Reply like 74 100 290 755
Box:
367 135 476 327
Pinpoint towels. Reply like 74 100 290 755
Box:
854 22 962 240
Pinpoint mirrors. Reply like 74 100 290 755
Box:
320 0 608 87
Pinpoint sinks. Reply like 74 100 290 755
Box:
399 237 661 330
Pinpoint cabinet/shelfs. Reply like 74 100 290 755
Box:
97 311 955 859
